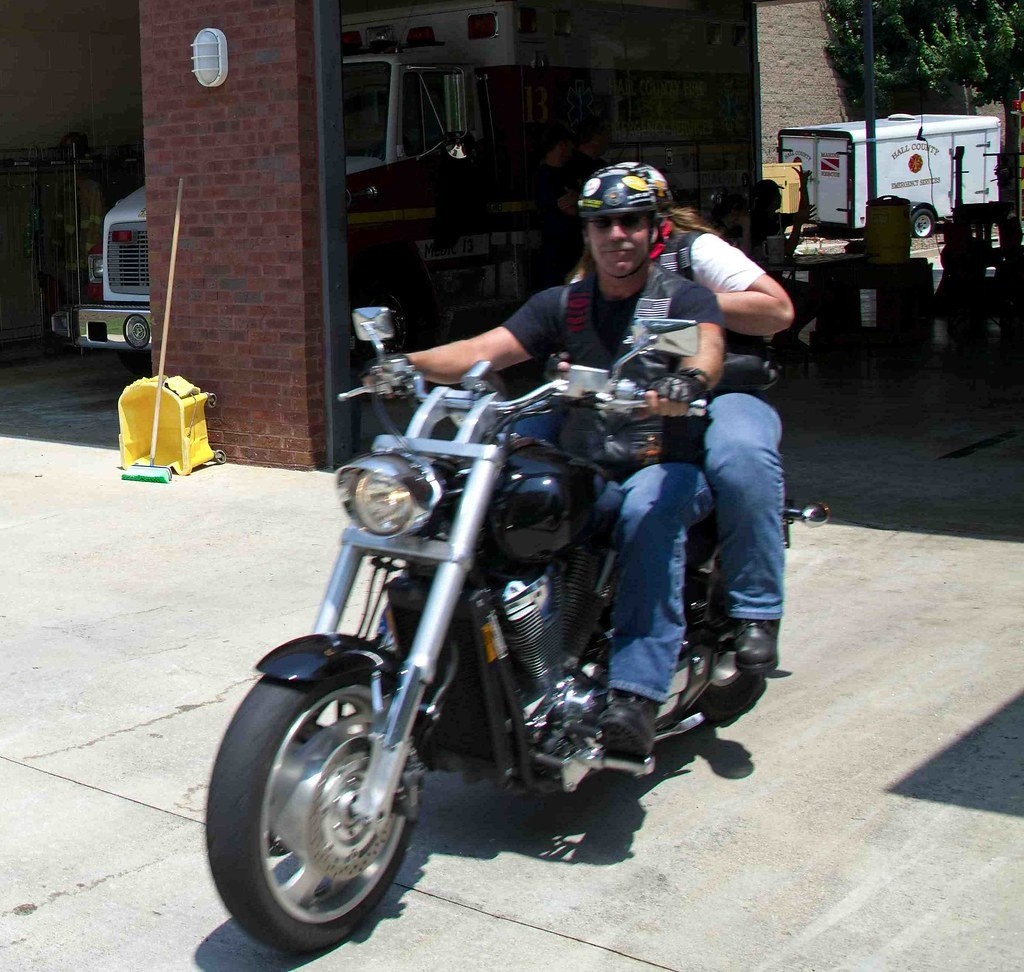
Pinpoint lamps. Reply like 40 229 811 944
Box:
191 28 228 88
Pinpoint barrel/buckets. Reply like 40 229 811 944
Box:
864 195 912 266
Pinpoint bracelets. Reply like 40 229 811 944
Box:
672 366 712 383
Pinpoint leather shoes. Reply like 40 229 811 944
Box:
598 699 659 759
736 621 779 673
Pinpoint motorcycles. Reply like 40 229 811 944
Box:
206 305 831 952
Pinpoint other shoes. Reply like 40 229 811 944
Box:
773 333 808 357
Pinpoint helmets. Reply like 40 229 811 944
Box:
577 161 672 216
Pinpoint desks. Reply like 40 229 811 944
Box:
743 253 869 380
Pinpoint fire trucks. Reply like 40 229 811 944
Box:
52 3 764 373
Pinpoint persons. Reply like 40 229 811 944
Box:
750 164 824 359
522 116 613 295
542 162 790 674
361 165 725 761
24 130 105 358
709 185 752 248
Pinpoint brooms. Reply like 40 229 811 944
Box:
121 177 184 484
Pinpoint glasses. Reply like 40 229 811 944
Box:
582 212 651 228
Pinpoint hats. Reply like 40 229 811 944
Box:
752 180 784 195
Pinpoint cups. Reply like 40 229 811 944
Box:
761 235 785 264
